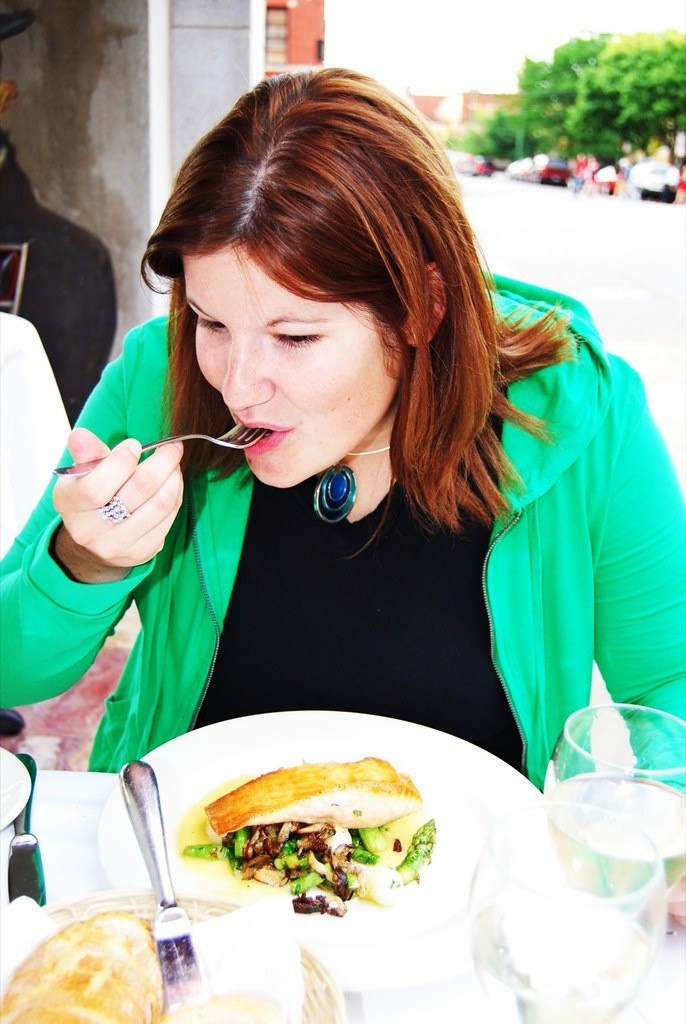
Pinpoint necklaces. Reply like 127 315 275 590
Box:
313 446 391 522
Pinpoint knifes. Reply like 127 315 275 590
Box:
119 761 207 1011
8 753 46 906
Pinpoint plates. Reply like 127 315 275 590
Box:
98 711 543 991
0 747 32 831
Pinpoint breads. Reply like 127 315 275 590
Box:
0 909 291 1024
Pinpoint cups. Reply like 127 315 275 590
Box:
544 704 686 912
467 805 667 1024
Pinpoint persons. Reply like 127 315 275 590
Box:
573 153 599 192
0 66 686 796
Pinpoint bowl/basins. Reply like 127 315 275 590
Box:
0 892 348 1024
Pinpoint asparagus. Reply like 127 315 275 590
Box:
178 819 437 896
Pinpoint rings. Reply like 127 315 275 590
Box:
98 496 131 524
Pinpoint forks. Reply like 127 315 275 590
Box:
50 422 270 478
